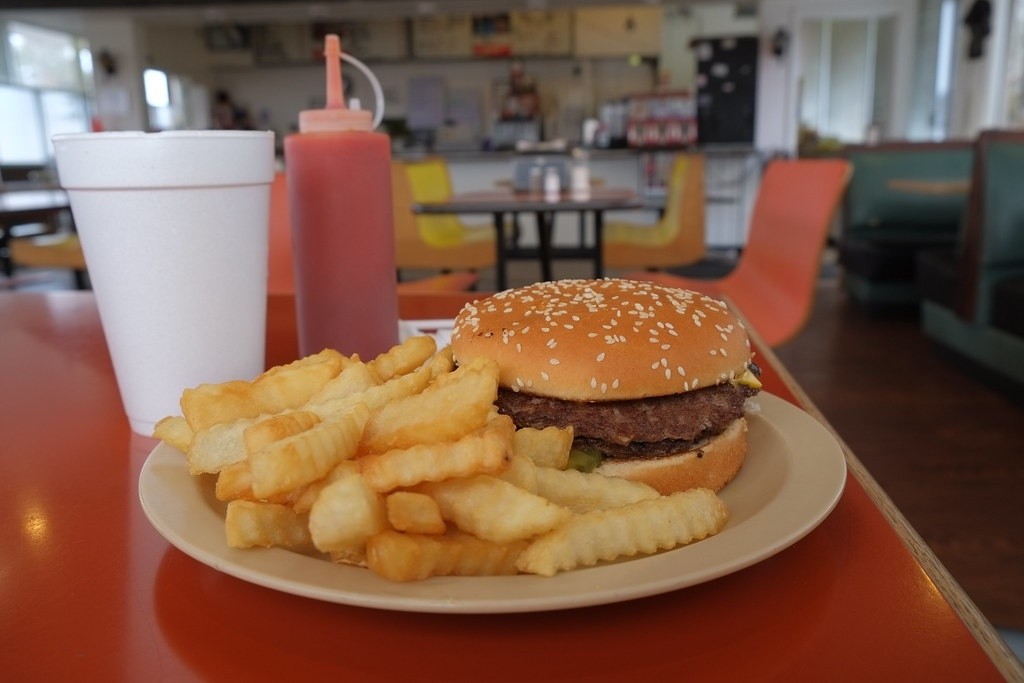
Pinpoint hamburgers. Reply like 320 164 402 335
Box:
450 280 762 496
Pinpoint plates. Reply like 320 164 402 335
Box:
137 390 848 612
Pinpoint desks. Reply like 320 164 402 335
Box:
412 184 642 288
0 284 1024 683
0 189 71 276
887 181 969 197
10 231 86 269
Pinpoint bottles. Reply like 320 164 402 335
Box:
569 151 591 203
527 166 543 203
282 33 399 368
542 165 562 203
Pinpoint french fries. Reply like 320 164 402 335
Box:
152 335 729 576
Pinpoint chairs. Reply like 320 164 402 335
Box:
621 152 861 351
603 151 707 272
393 161 515 272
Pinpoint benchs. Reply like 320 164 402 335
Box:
837 124 1024 391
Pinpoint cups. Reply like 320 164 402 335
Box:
51 126 276 440
